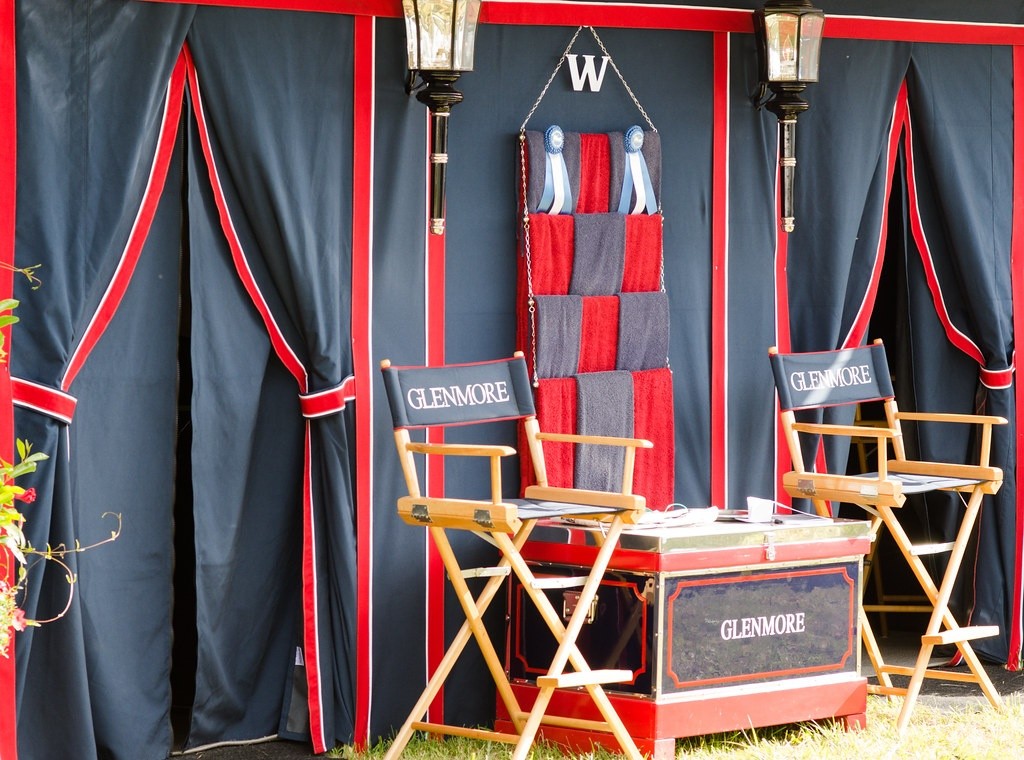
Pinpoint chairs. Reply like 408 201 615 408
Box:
381 350 655 760
769 339 1009 741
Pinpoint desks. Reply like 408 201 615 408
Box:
493 511 874 760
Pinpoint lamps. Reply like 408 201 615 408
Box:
750 0 826 231
401 0 482 235
